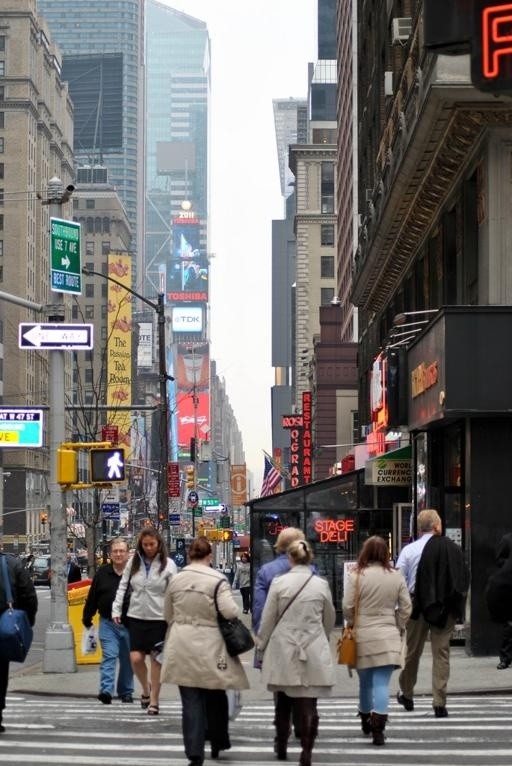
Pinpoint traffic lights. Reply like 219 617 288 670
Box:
223 530 232 540
91 449 125 482
185 468 195 488
40 513 47 524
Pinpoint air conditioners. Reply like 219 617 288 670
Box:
391 17 412 46
383 71 394 97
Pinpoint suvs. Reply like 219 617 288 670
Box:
31 554 78 584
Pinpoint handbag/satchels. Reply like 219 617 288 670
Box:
217 611 255 656
2 601 33 663
336 627 356 665
121 590 130 624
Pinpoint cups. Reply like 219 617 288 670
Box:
184 354 204 383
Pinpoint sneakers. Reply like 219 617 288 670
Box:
188 749 219 765
98 692 112 704
496 662 509 669
123 695 133 703
435 706 448 717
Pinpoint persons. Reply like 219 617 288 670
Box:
5 551 113 590
159 537 242 765
231 551 253 614
250 538 338 764
79 535 139 708
250 527 321 640
341 535 415 747
0 544 41 734
485 529 511 670
391 509 470 718
107 529 181 716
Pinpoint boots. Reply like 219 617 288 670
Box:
358 712 387 745
274 717 291 761
299 716 319 765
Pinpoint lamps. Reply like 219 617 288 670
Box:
373 308 441 362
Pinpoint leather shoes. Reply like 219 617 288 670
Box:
397 689 414 711
141 683 159 715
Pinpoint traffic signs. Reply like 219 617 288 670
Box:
50 270 80 292
50 219 80 274
18 322 93 348
